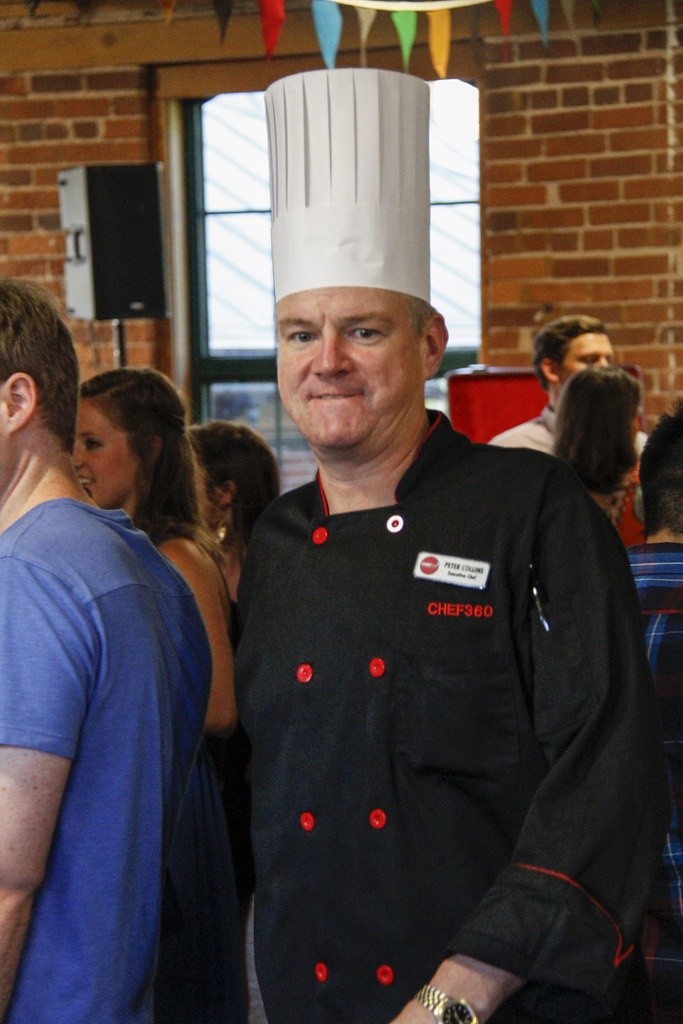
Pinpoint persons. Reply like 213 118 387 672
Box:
552 365 646 547
77 368 242 1023
236 66 657 1024
625 403 682 1022
186 420 281 1024
0 283 212 1023
489 315 617 469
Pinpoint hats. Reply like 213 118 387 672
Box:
263 67 431 305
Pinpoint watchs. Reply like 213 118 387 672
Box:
415 985 481 1024
